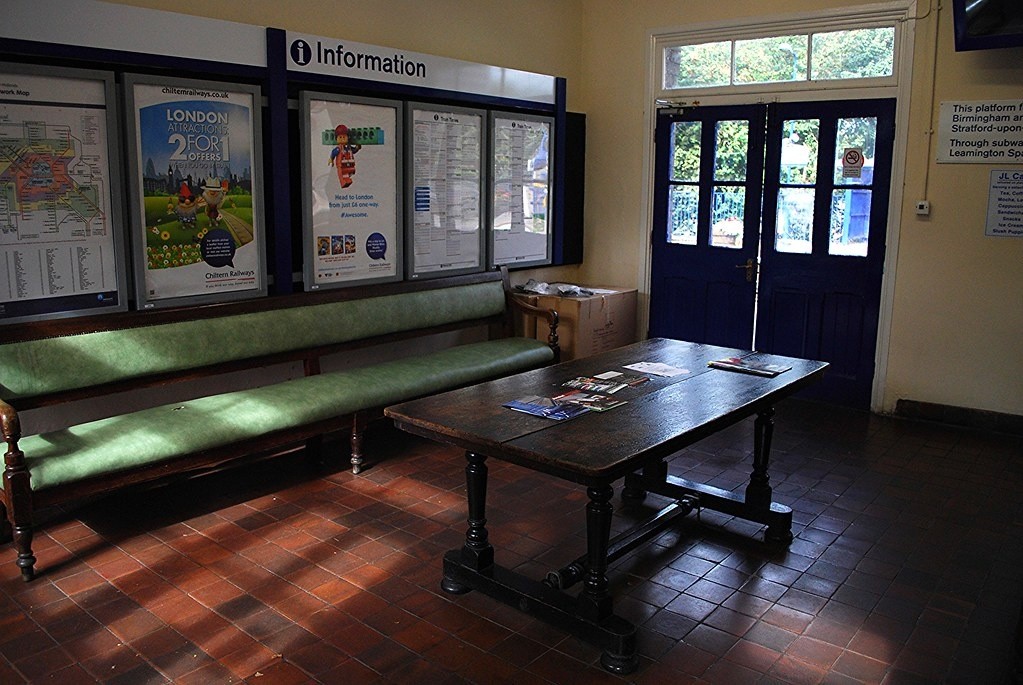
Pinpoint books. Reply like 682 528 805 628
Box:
708 350 793 377
502 371 650 421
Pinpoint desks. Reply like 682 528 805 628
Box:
383 334 830 677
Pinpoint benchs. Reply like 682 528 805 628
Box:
0 267 559 583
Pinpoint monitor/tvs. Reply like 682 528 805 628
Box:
952 0 1023 52
